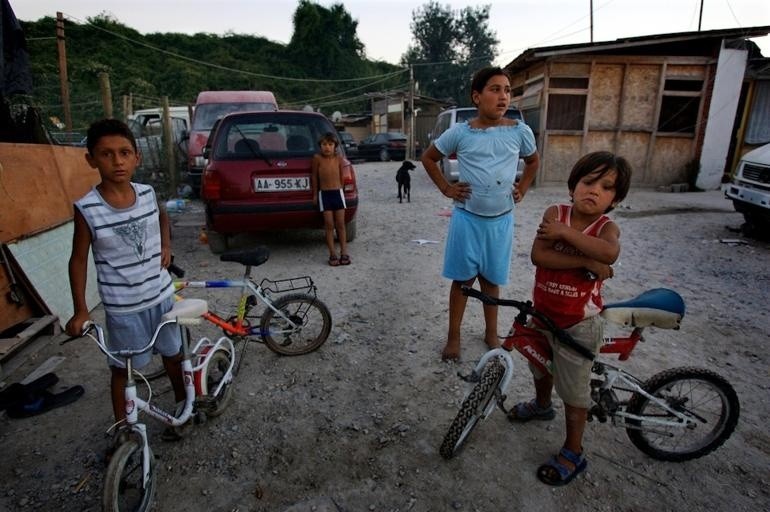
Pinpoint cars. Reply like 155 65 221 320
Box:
338 132 359 161
355 132 421 163
201 110 358 249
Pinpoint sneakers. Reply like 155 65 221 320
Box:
162 398 195 440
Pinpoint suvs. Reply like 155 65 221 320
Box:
724 143 770 237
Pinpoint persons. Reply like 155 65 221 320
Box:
508 151 633 486
311 132 352 265
421 68 540 359
65 119 194 468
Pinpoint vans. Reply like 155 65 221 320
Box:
427 105 532 182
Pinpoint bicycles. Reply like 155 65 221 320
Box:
438 283 741 463
166 248 333 377
59 297 235 511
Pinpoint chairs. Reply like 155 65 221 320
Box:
287 135 310 150
234 139 259 153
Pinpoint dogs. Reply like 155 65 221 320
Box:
395 160 417 203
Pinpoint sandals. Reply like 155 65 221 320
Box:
508 398 555 422
329 256 338 266
340 255 350 265
538 445 587 486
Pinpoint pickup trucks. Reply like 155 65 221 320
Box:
127 107 194 152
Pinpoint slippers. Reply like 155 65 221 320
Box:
0 373 58 409
6 386 84 418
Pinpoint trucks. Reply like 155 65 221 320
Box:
180 91 296 197
